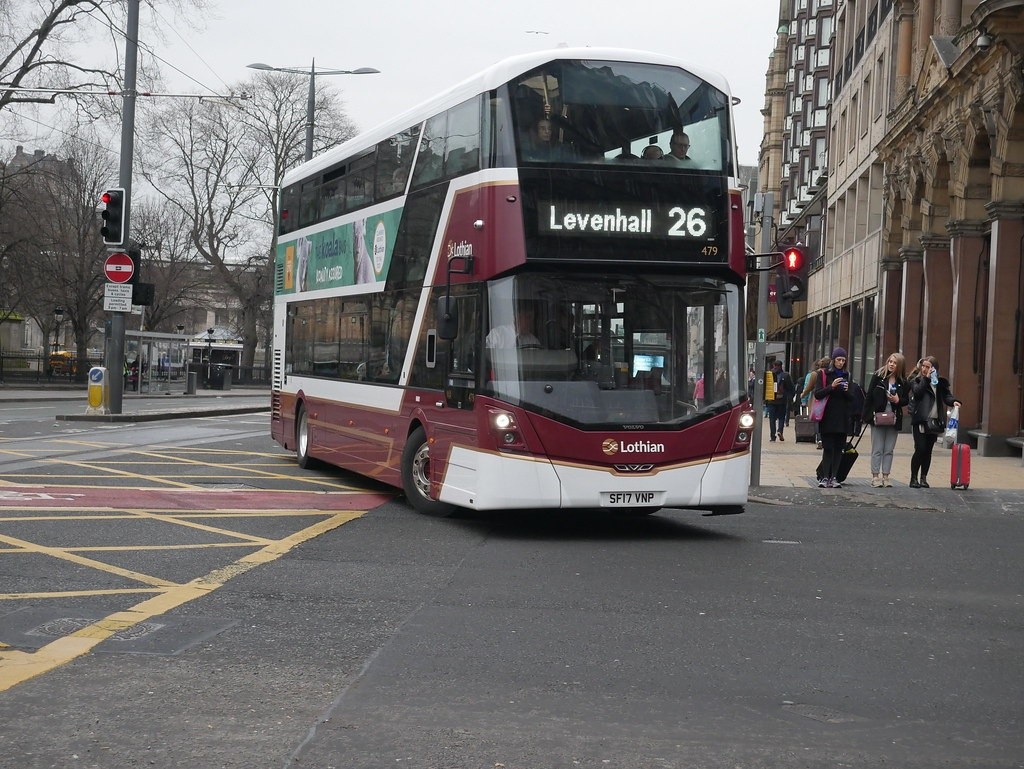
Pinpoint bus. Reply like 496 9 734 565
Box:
269 45 801 516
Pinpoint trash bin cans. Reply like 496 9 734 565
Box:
210 363 232 389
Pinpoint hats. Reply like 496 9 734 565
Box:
773 360 783 365
832 347 847 359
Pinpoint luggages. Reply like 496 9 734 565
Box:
794 415 817 444
949 443 971 491
815 419 869 483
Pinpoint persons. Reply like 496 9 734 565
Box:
847 379 866 436
123 355 138 392
391 167 408 192
525 117 573 163
861 353 910 490
583 336 603 361
748 357 832 450
613 132 696 166
814 348 854 489
906 356 962 489
46 364 57 383
543 312 577 350
687 371 731 410
486 305 545 353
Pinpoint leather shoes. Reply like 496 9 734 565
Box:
910 476 921 488
919 475 930 488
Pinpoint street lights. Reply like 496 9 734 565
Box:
206 327 215 364
246 58 380 164
176 323 185 362
55 305 65 355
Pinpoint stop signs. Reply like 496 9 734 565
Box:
101 252 136 284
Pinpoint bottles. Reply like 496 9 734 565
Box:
931 366 938 384
890 384 897 396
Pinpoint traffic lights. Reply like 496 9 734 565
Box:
101 188 126 245
774 241 809 302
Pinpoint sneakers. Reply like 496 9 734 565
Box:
870 478 883 487
882 478 892 488
828 476 841 487
818 478 829 488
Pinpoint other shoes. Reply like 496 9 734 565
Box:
770 438 776 443
777 432 785 442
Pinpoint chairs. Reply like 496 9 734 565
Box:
582 344 676 394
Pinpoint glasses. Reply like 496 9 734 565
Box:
672 142 691 149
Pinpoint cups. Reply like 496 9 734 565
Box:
840 379 847 389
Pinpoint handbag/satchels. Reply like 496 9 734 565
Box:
809 370 830 420
945 406 959 442
874 411 897 426
929 418 944 433
